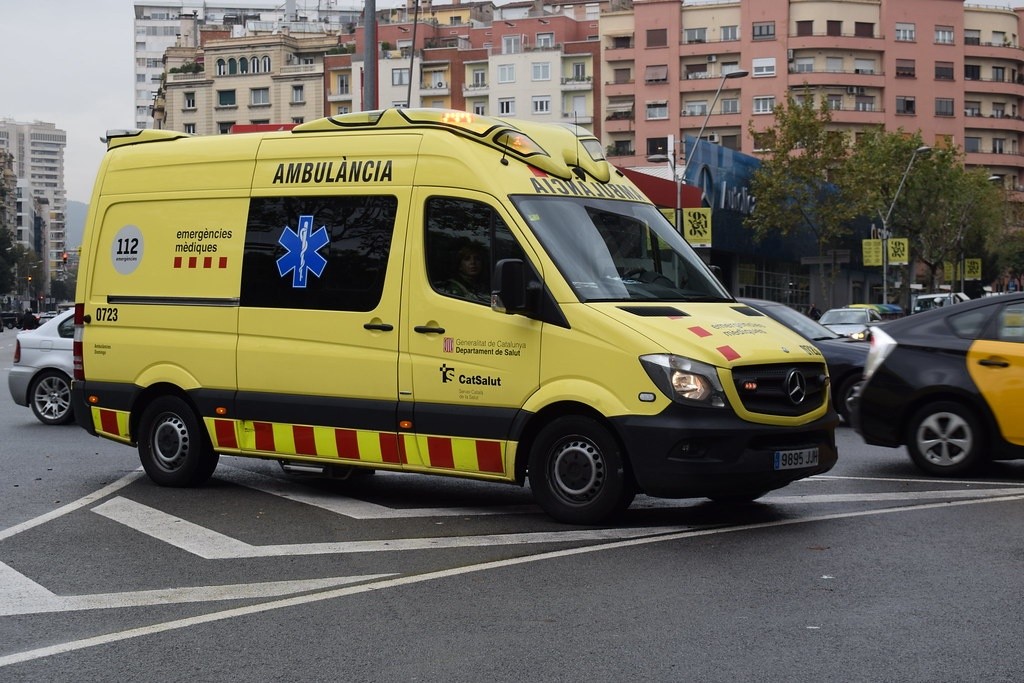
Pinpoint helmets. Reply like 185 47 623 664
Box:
25 308 33 313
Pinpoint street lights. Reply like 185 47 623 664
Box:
646 69 749 290
960 176 1003 291
877 145 933 304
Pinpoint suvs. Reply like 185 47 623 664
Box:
1 311 23 329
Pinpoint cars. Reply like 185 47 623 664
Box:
732 296 872 429
7 304 76 424
818 307 883 341
31 311 57 328
848 290 1024 475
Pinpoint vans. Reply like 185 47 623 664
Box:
913 292 971 314
845 303 906 321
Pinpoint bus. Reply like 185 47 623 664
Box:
57 303 75 315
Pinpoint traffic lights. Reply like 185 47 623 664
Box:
29 275 32 284
63 248 67 264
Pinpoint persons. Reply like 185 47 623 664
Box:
432 246 491 305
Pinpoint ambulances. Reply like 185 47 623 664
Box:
74 107 842 524
